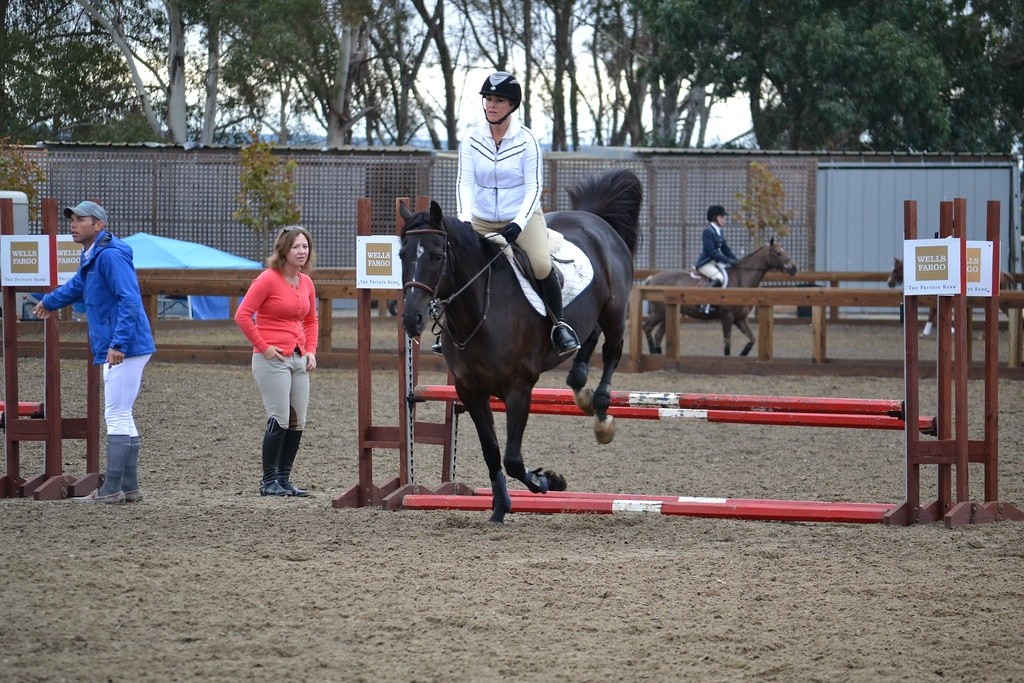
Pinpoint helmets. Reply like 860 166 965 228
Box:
479 72 522 105
707 205 727 217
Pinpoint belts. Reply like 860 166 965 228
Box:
294 348 300 352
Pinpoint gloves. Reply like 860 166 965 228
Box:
728 258 737 268
497 223 522 244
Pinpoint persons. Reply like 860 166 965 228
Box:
235 226 320 499
34 200 155 504
696 206 738 317
455 70 577 352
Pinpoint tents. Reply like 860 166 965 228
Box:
121 232 261 319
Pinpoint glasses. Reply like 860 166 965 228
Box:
284 226 304 232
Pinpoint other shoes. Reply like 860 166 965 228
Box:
71 487 127 504
124 488 143 501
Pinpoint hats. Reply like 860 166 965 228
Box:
63 201 108 226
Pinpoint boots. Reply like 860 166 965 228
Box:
277 429 310 497
258 415 293 496
697 278 722 314
534 265 578 352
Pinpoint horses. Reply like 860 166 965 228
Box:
886 256 1023 341
396 167 644 527
641 234 799 357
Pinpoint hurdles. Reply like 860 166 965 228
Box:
328 195 1023 529
0 194 103 504
